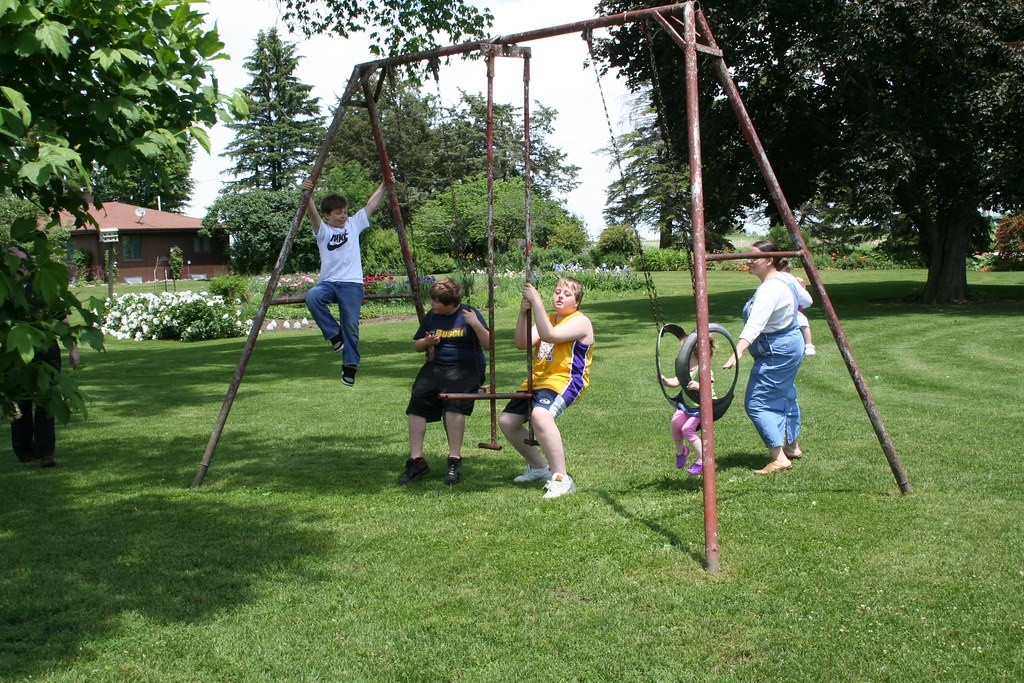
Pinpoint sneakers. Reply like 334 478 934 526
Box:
330 326 345 353
542 472 576 499
513 465 550 482
340 365 358 386
396 457 431 487
444 453 463 486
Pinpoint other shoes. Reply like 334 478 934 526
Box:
804 344 816 355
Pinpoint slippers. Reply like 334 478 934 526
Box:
762 462 793 475
782 447 803 459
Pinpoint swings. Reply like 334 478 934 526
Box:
580 19 742 434
382 56 491 400
432 43 543 454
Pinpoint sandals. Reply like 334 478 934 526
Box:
688 460 703 475
676 446 690 469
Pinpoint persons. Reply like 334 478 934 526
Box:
497 277 596 501
655 334 723 474
397 276 490 486
301 170 396 387
722 239 816 474
